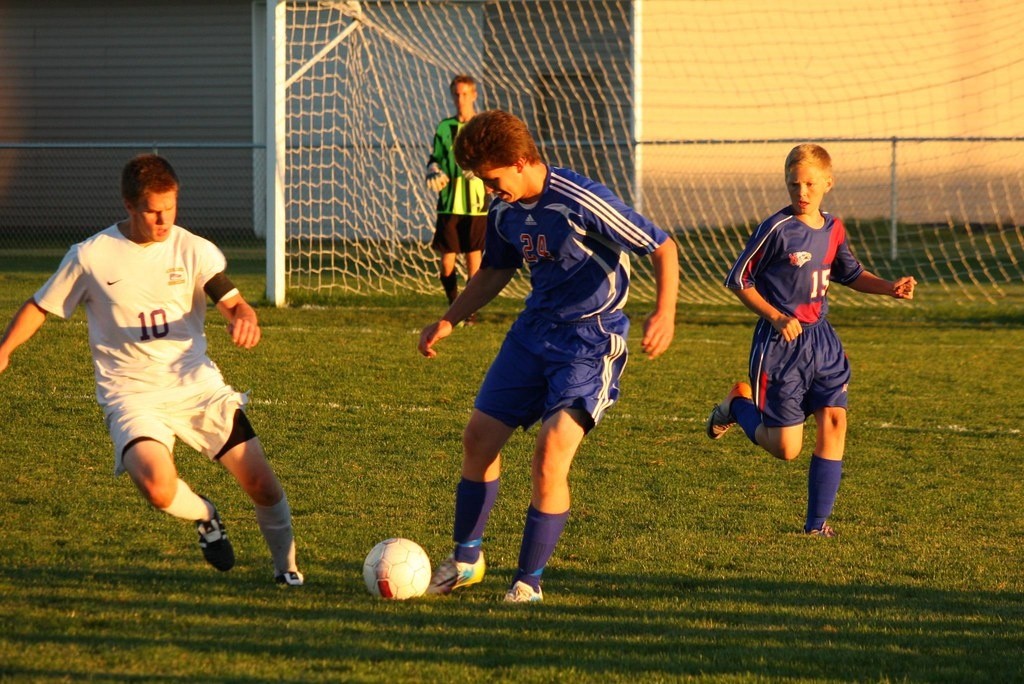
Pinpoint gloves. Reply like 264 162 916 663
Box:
426 162 450 192
462 170 474 179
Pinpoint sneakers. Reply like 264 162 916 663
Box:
502 579 544 602
454 320 464 327
803 521 838 537
464 313 476 327
706 382 752 440
195 495 235 572
422 549 487 595
274 570 305 587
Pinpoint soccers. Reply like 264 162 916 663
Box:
363 537 433 602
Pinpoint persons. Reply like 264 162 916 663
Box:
706 143 917 535
424 76 495 327
0 153 307 593
417 108 679 605
